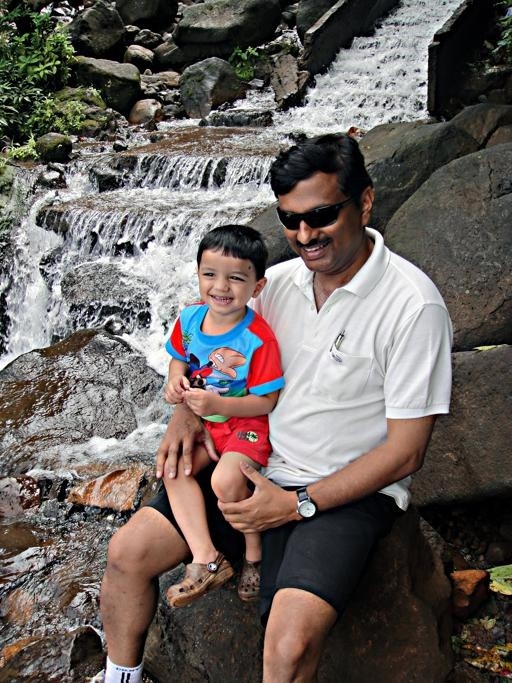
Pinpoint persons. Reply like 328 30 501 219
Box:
160 224 286 607
90 130 455 683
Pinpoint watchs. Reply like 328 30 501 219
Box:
295 485 318 520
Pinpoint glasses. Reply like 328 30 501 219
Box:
276 198 351 230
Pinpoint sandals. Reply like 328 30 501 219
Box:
238 553 263 602
167 553 235 608
